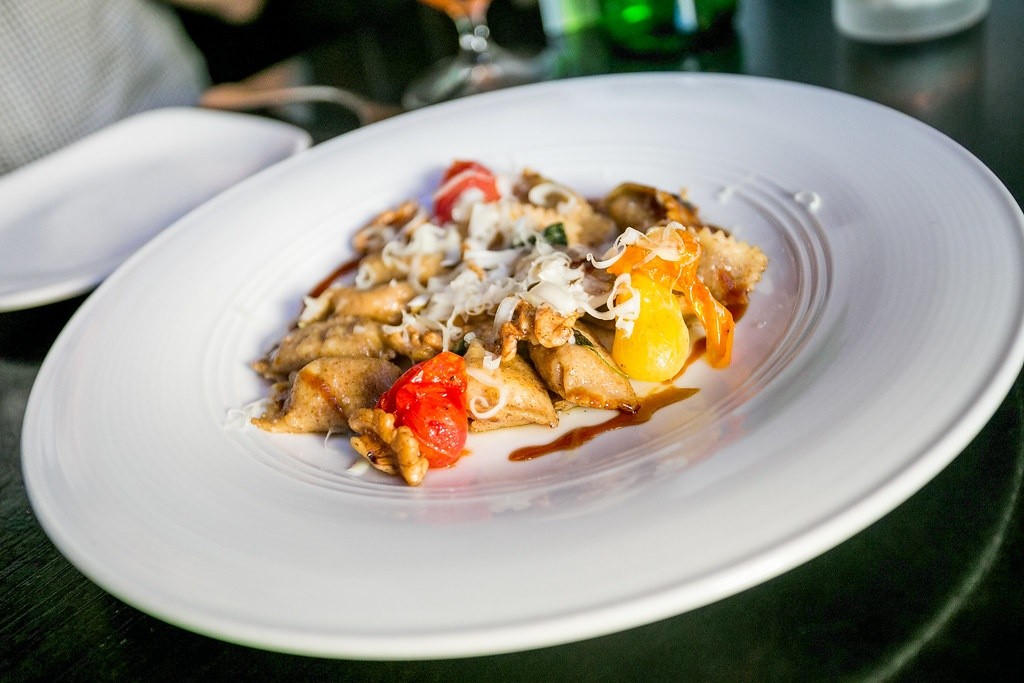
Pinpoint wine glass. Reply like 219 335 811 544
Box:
399 1 549 111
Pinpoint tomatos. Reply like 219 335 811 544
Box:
369 351 469 468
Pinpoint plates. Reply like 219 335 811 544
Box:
0 106 313 312
22 73 1024 659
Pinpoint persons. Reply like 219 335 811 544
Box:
0 0 205 180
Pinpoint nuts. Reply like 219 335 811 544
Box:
382 324 457 362
348 407 429 488
